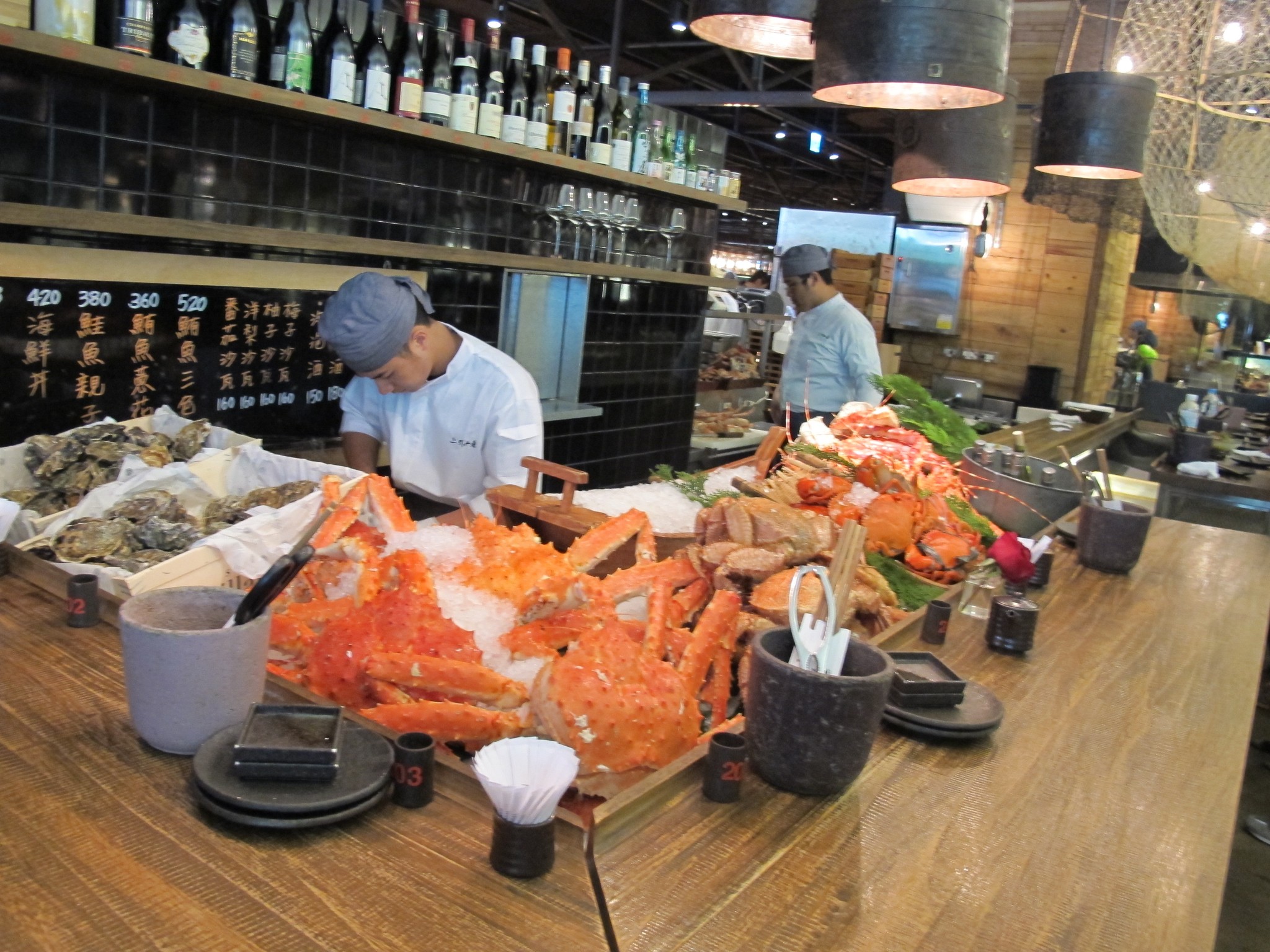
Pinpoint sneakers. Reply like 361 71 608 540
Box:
1244 668 1270 847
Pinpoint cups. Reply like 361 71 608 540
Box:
1076 497 1152 574
744 626 895 795
1005 548 1055 596
1197 416 1223 433
921 600 952 645
395 733 435 807
118 586 271 754
66 574 99 628
491 808 557 880
956 569 999 619
1166 430 1213 466
703 731 747 805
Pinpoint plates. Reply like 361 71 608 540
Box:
190 702 398 828
1231 413 1269 450
1229 455 1270 466
1217 463 1255 477
882 650 1003 738
1056 522 1077 544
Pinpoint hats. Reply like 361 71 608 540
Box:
317 272 436 374
1133 321 1147 332
781 244 839 276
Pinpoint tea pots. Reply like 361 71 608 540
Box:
984 591 1040 652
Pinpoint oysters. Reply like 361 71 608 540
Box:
1 418 319 574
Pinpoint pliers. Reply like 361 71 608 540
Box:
788 565 836 676
1082 471 1105 507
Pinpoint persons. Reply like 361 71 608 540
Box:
736 270 772 312
316 271 543 522
774 243 884 445
1127 321 1160 359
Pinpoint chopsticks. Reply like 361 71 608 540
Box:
1096 448 1113 501
1057 446 1092 501
288 498 336 558
812 517 867 636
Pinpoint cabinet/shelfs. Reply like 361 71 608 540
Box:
4 26 747 289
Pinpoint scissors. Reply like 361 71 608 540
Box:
1169 415 1188 432
221 545 314 629
1199 400 1231 420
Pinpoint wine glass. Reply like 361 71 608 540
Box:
522 180 686 271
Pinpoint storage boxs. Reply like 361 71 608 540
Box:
0 402 369 631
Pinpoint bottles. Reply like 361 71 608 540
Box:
30 0 698 188
974 431 1056 487
1178 390 1219 428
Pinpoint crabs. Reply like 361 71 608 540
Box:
245 472 741 777
673 498 917 641
783 379 986 585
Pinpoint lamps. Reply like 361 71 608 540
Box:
689 0 1157 196
